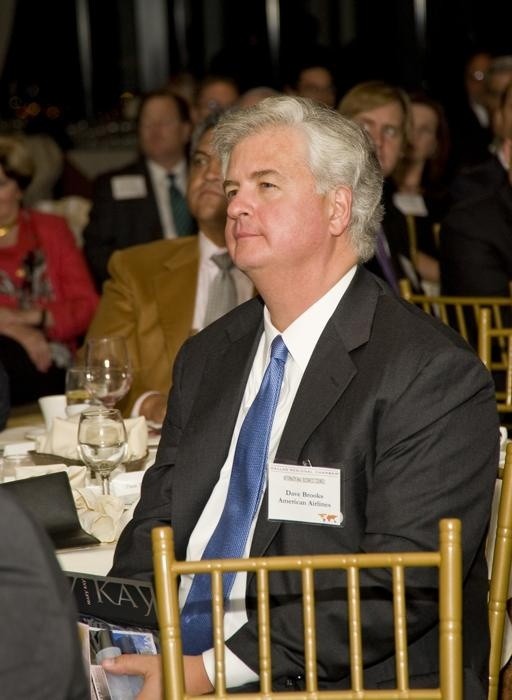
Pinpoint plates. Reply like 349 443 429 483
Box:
0 404 150 477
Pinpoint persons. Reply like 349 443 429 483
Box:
0 488 93 699
0 56 512 422
102 96 499 699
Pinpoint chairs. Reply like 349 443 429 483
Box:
393 211 512 699
150 513 465 700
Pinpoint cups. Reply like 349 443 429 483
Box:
1 454 36 484
39 394 71 431
65 365 113 410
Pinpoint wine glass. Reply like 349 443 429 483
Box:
77 407 128 498
84 335 135 406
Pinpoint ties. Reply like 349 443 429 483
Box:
166 171 199 236
205 252 237 329
180 334 287 654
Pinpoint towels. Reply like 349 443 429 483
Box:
3 465 142 546
19 411 150 463
